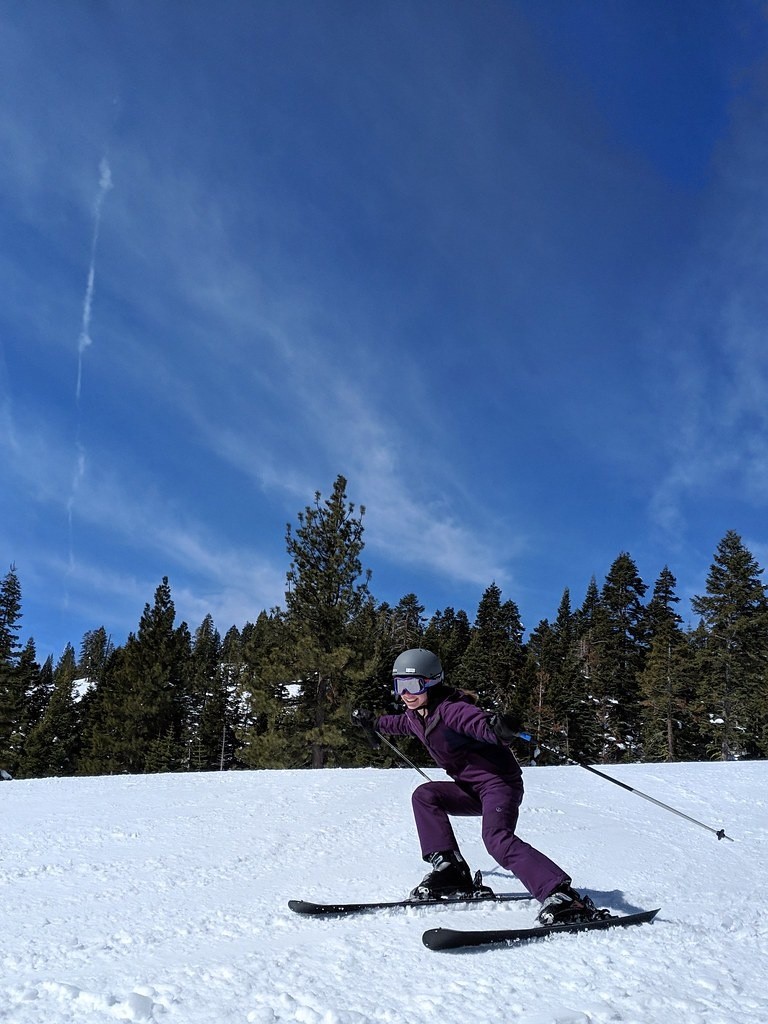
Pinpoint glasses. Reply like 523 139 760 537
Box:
393 677 441 695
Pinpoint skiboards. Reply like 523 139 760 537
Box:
284 898 663 952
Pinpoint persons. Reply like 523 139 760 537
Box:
349 650 614 927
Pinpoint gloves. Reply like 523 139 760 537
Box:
488 713 526 742
350 708 380 731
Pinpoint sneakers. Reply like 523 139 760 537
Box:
408 848 496 901
535 879 612 927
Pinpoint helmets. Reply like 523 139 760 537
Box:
392 648 442 678
